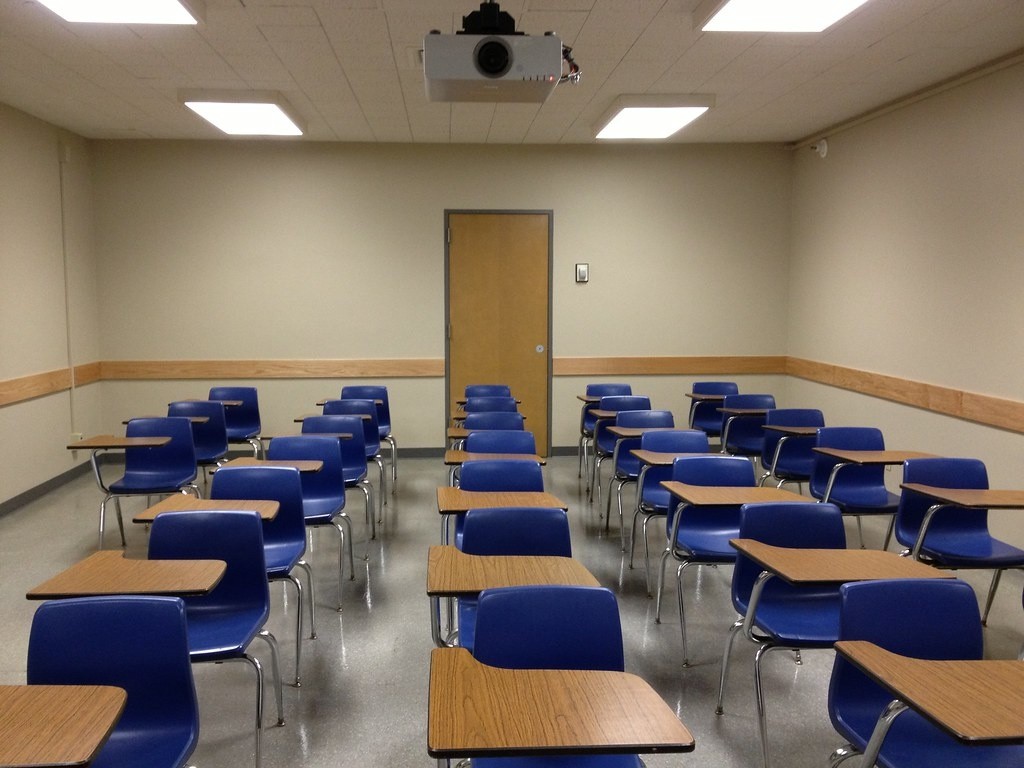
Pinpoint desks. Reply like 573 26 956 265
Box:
812 447 944 504
833 640 1024 768
899 484 1024 566
435 487 568 545
209 457 324 474
169 400 243 406
604 426 695 481
294 415 372 421
686 393 724 429
316 399 384 406
131 495 281 522
261 432 354 441
455 398 521 403
717 408 768 455
427 648 697 768
577 396 601 438
26 549 227 596
629 449 729 516
589 409 616 458
447 428 471 439
0 685 129 768
728 539 956 643
762 425 819 481
660 481 819 560
453 412 527 419
66 434 172 493
427 545 604 647
122 415 209 424
444 450 547 466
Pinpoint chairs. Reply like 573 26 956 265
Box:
0 382 1024 768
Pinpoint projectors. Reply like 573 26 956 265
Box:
423 34 563 104
